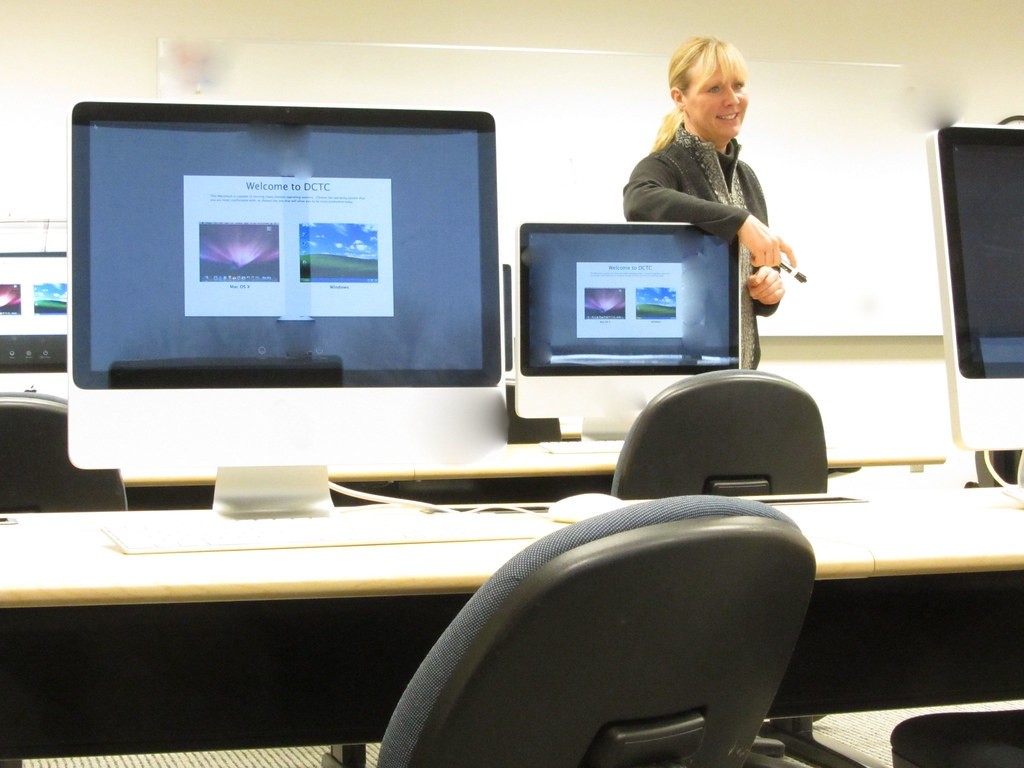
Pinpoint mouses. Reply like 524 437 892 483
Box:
549 492 623 523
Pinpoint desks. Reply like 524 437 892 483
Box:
0 446 1024 768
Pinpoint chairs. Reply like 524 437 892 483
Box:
0 390 129 511
376 494 816 767
610 367 829 498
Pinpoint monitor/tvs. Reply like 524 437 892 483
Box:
928 124 1024 507
0 102 743 556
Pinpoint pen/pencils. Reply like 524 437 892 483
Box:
777 258 809 285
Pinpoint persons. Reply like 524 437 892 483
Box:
624 37 796 371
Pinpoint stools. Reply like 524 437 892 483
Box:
890 708 1024 768
